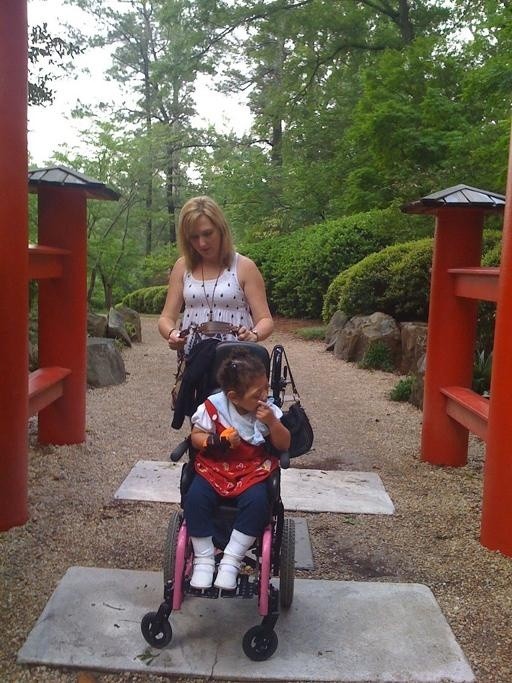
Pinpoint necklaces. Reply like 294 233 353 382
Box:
202 262 222 321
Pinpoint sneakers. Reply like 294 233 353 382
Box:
215 557 240 591
190 556 214 589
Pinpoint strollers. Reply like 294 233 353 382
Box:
139 338 296 662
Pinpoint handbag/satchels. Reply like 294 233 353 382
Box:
263 401 315 456
170 356 198 411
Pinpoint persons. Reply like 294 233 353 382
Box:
182 346 291 591
157 195 275 367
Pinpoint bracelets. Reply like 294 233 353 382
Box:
168 328 175 337
251 330 260 342
